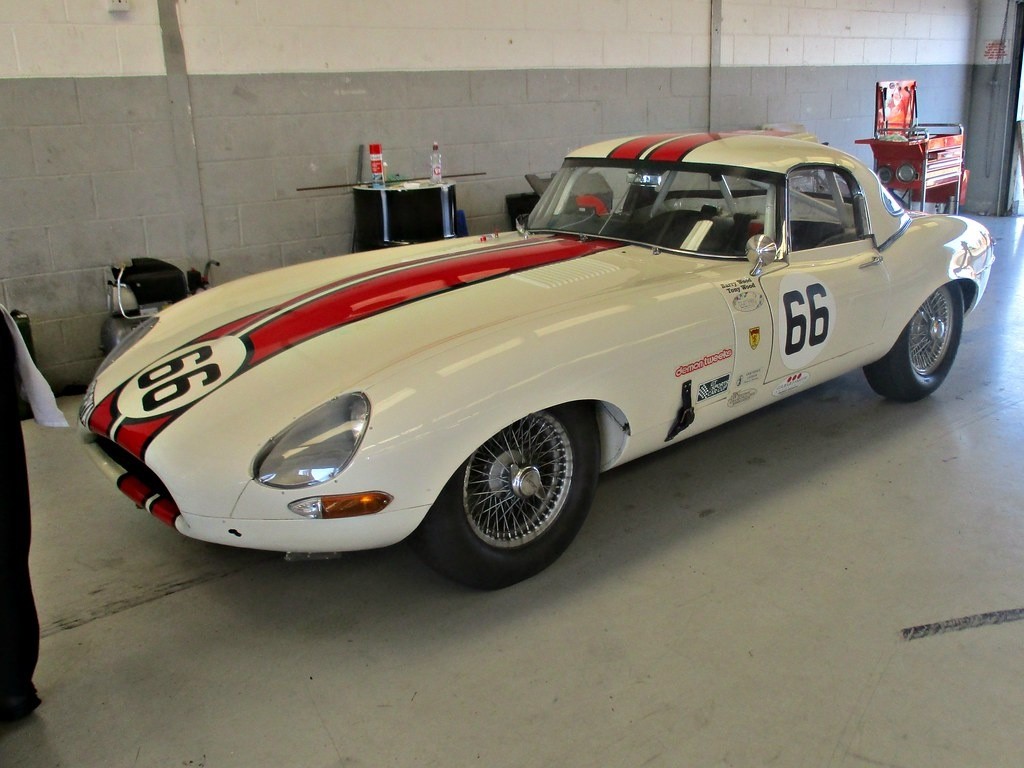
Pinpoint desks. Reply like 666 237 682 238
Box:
351 175 461 254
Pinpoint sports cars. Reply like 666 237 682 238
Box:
78 128 999 595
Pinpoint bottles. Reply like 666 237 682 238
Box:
369 143 387 188
430 142 441 183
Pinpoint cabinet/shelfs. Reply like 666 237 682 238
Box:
870 124 971 214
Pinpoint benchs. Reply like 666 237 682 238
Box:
650 208 845 256
